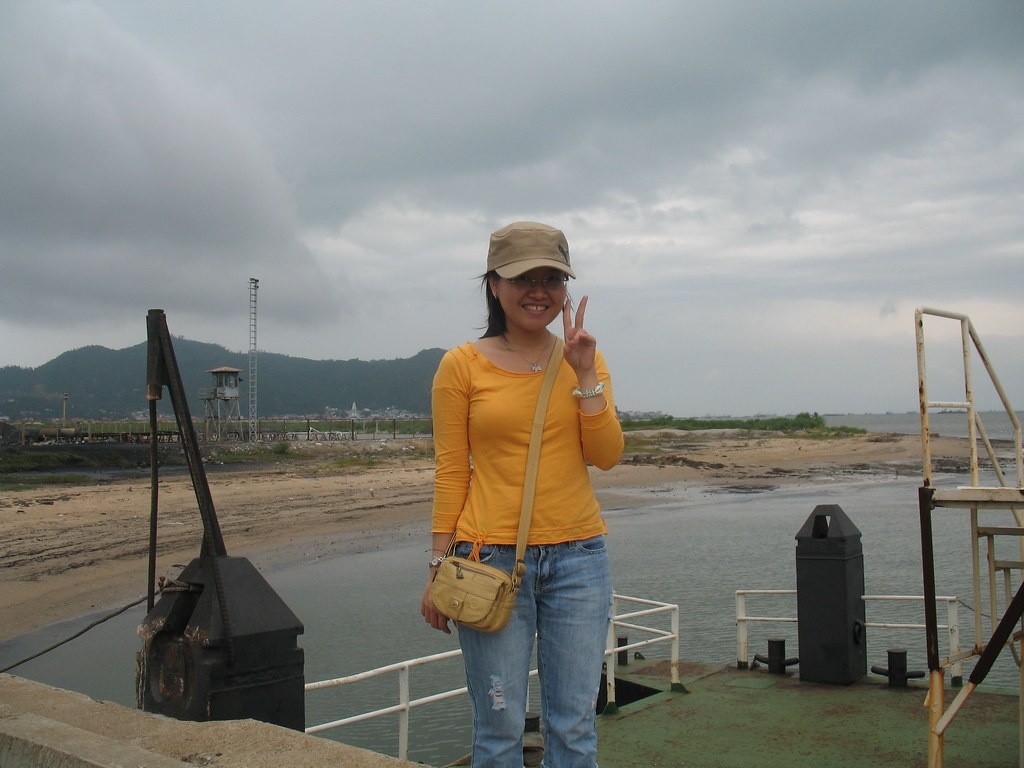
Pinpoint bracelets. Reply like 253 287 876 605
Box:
572 382 605 398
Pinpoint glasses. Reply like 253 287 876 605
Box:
504 271 570 292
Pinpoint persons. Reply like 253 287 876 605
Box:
420 221 624 768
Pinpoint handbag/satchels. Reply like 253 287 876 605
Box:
428 555 520 633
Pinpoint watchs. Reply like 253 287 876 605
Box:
428 556 443 568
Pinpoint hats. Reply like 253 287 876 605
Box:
486 222 576 279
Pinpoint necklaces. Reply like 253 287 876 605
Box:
502 329 551 372
